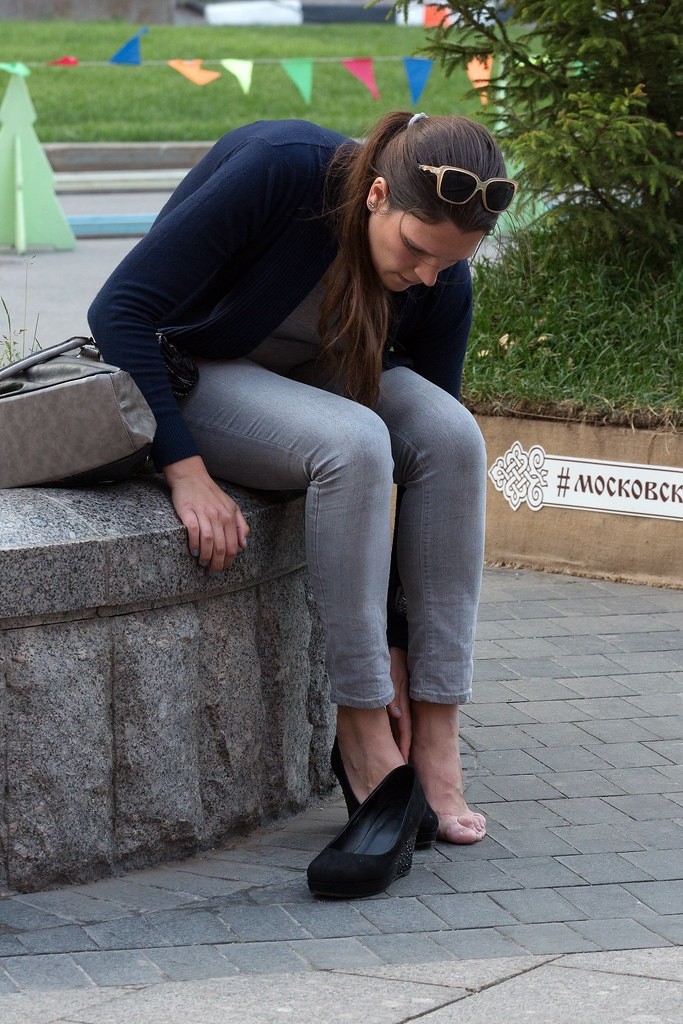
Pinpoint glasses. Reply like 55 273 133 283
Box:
419 163 521 214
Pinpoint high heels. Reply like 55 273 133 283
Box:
331 734 439 850
306 765 426 898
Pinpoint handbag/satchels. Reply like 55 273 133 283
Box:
0 337 157 489
114 334 196 480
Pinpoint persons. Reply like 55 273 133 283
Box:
86 108 518 844
